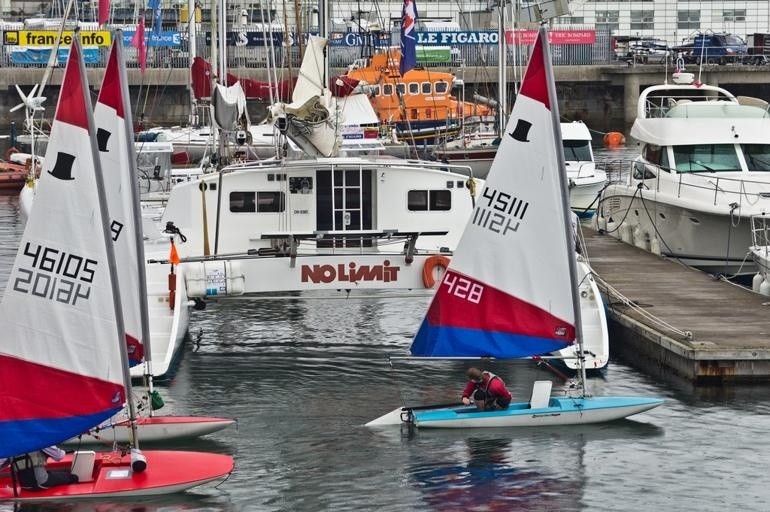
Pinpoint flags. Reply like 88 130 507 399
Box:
398 1 418 79
148 0 163 39
130 16 147 75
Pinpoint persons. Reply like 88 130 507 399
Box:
12 445 79 491
460 364 511 412
